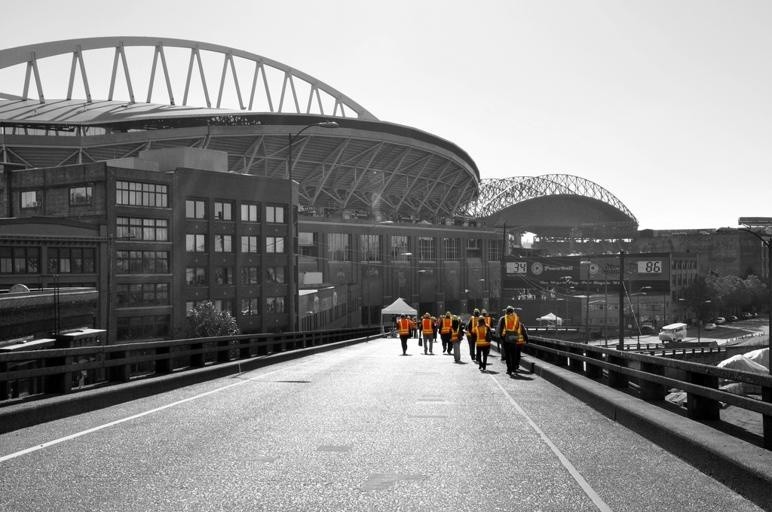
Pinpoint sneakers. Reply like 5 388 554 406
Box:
403 348 519 374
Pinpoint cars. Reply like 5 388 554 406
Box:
704 312 759 330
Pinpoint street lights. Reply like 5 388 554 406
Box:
288 121 340 327
365 221 394 327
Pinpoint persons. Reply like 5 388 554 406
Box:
395 305 530 376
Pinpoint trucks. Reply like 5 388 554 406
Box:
659 322 687 344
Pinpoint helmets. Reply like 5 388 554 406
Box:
401 306 515 322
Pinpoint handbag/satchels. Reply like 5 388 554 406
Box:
419 338 422 346
505 331 518 345
485 327 492 342
